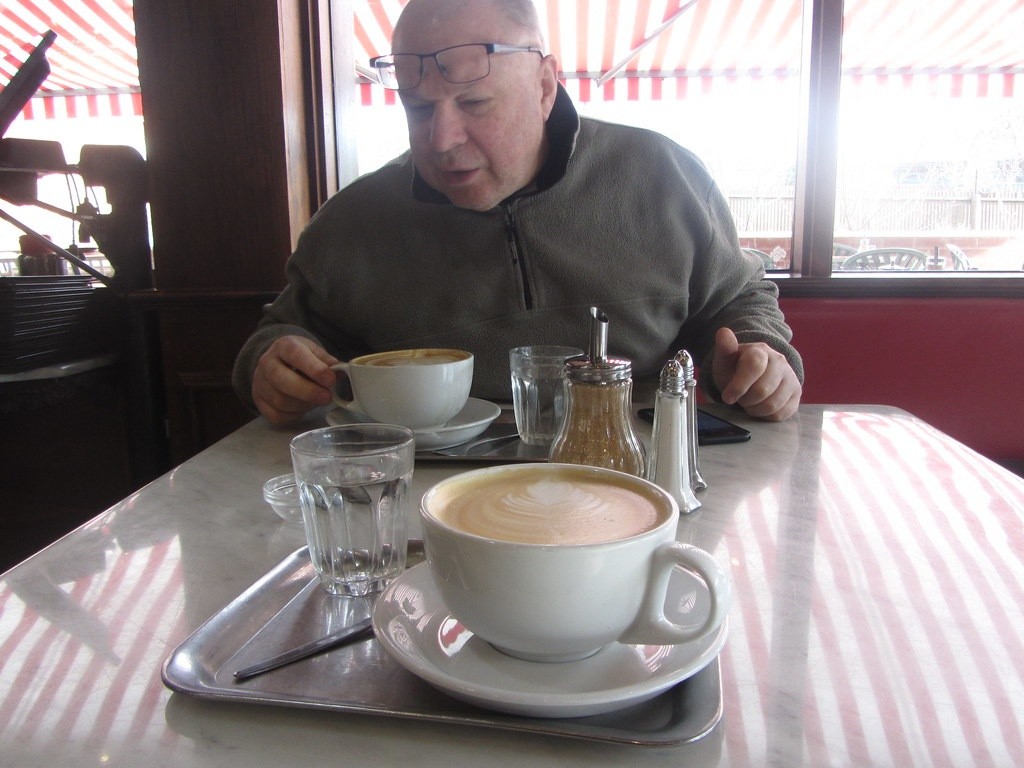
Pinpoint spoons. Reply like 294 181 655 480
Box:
431 433 519 456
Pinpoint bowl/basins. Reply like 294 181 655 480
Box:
263 472 302 523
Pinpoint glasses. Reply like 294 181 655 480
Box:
370 43 545 90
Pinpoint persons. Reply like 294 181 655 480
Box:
233 0 804 425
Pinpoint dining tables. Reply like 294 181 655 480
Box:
1 403 1024 768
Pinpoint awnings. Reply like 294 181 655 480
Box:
0 1 1023 118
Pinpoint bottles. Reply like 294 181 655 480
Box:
548 306 648 480
648 359 700 514
675 349 708 492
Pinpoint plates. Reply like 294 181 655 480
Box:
326 397 501 452
372 561 726 718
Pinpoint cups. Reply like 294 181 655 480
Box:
509 346 583 446
329 349 474 427
289 424 415 597
420 462 726 662
